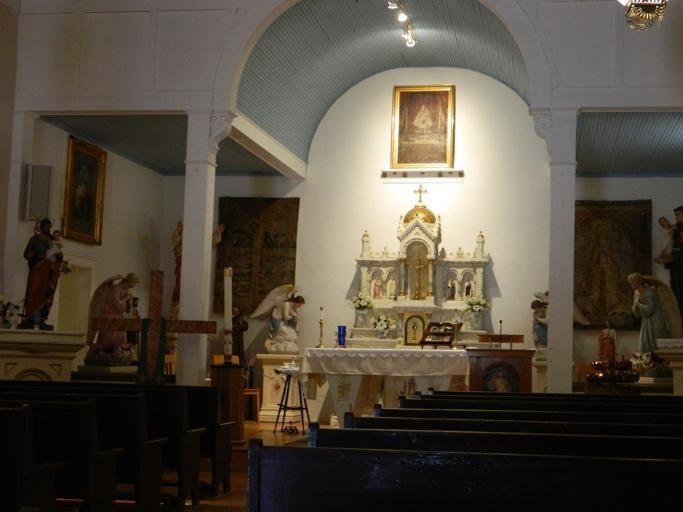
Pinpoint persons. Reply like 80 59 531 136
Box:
628 272 669 352
659 217 678 256
87 273 140 346
272 296 305 342
122 297 138 344
598 320 617 353
657 206 683 327
46 231 63 262
17 216 63 330
232 305 248 363
532 301 549 346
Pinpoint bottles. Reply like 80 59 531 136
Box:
338 325 346 346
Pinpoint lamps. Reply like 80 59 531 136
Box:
388 0 399 11
616 0 667 31
398 8 416 47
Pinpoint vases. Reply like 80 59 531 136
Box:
356 310 363 328
473 304 481 312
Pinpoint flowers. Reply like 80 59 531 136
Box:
368 315 391 337
466 296 487 306
0 295 26 329
352 294 374 312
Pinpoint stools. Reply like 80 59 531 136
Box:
272 364 311 435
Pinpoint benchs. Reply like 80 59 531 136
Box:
0 380 239 512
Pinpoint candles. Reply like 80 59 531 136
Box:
224 266 232 361
320 306 323 320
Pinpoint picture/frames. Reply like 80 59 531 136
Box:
63 135 107 246
212 197 299 315
574 199 652 330
390 85 455 172
